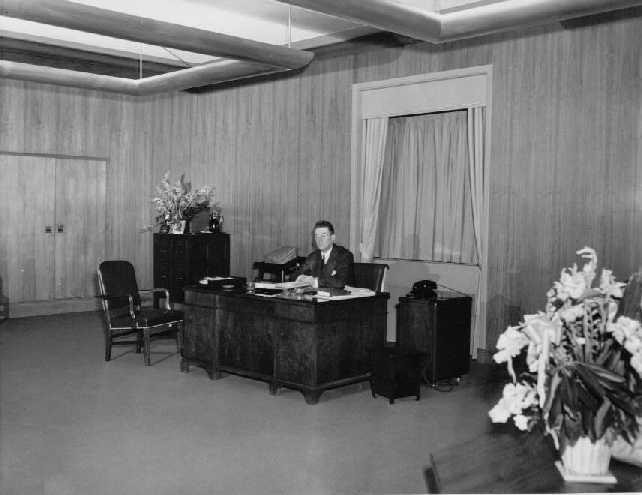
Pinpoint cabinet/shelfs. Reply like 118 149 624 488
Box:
150 233 229 313
395 297 473 392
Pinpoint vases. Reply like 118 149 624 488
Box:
551 425 616 483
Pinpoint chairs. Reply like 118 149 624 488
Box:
96 256 186 369
349 260 390 291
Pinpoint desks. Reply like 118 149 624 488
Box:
180 278 392 406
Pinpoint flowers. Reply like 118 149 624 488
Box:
489 247 641 438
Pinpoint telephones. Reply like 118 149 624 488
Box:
413 279 438 299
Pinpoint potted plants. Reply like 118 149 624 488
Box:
136 170 217 234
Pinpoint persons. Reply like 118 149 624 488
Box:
291 219 357 290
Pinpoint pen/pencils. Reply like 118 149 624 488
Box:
281 269 284 283
